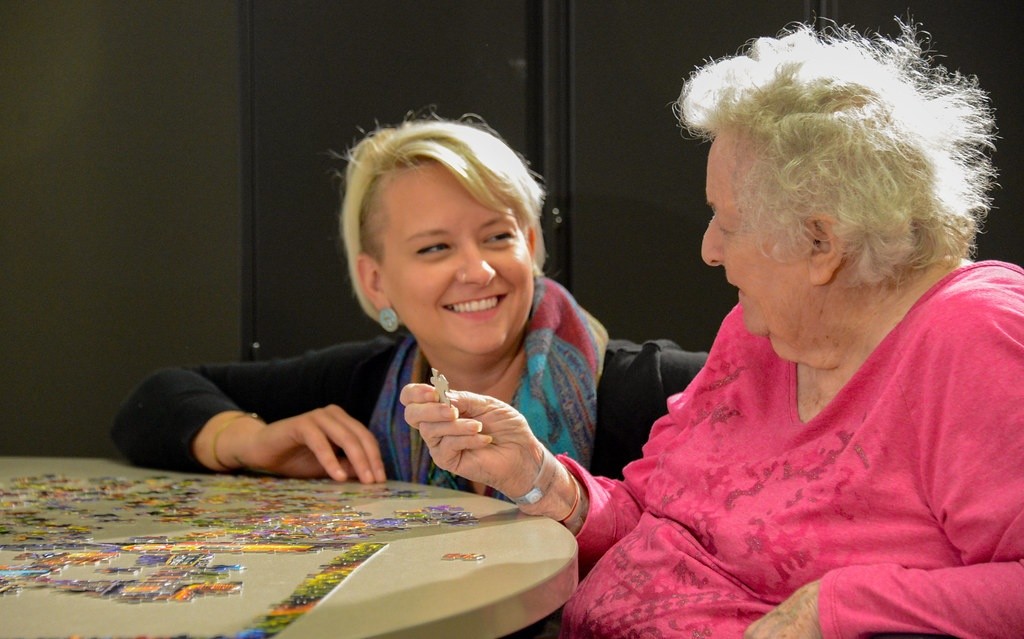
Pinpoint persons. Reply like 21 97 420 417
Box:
108 123 709 504
398 26 1024 639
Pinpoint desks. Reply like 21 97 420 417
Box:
0 454 577 639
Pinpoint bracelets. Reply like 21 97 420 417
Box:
509 447 582 528
214 413 262 467
